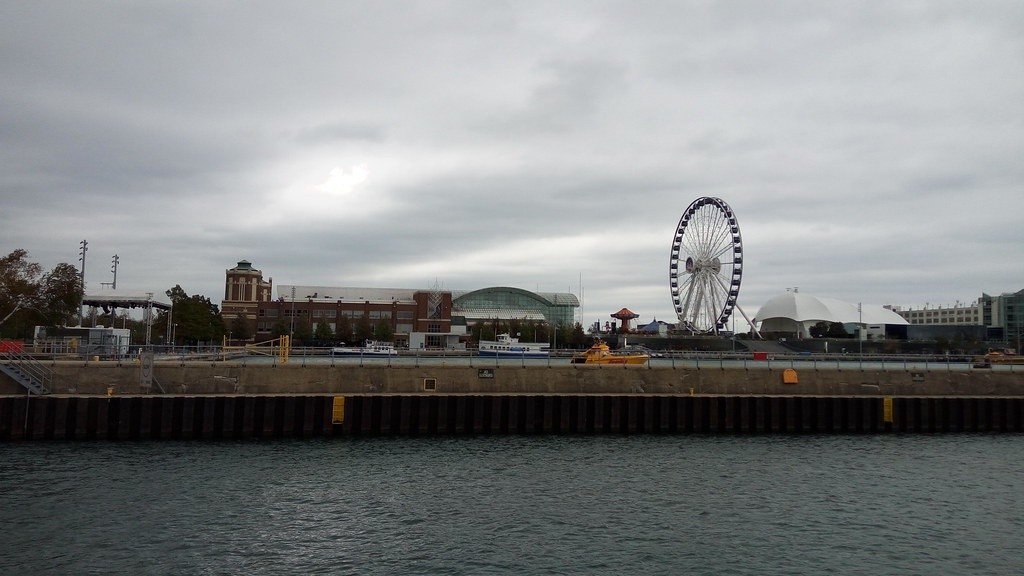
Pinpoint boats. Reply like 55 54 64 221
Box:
477 332 550 359
570 336 652 364
330 345 398 358
972 347 1024 367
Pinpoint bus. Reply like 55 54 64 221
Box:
32 324 131 357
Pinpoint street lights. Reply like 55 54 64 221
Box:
110 254 120 289
171 323 178 353
857 301 864 368
289 287 296 351
78 240 89 295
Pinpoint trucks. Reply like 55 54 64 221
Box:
365 339 394 348
